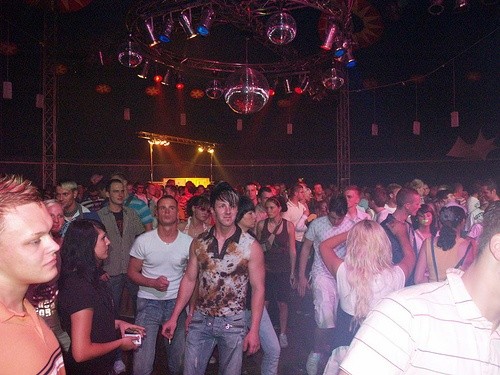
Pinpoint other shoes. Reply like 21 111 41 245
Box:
114 360 126 374
304 303 315 316
306 349 320 375
278 333 288 348
296 302 305 314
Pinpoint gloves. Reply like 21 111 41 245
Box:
307 213 317 223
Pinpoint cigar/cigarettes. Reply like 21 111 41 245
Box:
169 338 171 344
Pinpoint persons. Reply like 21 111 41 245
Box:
0 172 500 375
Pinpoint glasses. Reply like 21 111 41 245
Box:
195 206 211 214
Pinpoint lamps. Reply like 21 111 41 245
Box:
342 46 358 69
160 69 171 87
137 60 151 79
319 20 340 51
196 4 217 37
151 62 163 82
294 75 309 95
157 12 177 43
142 16 160 48
268 78 280 96
178 8 198 41
283 76 293 94
175 71 185 90
